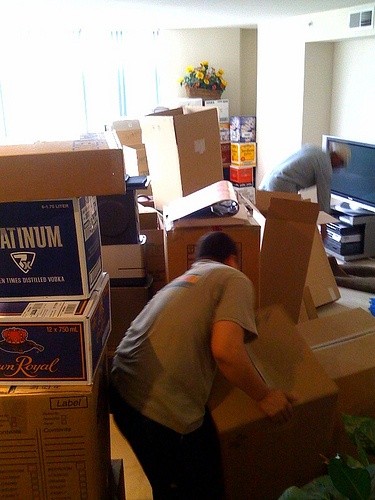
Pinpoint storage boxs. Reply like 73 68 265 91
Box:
0 97 375 500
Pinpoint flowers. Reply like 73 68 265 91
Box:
177 61 227 92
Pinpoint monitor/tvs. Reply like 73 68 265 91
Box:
321 135 375 215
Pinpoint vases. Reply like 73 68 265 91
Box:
183 85 223 99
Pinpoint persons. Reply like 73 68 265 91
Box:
259 141 351 240
109 231 300 500
